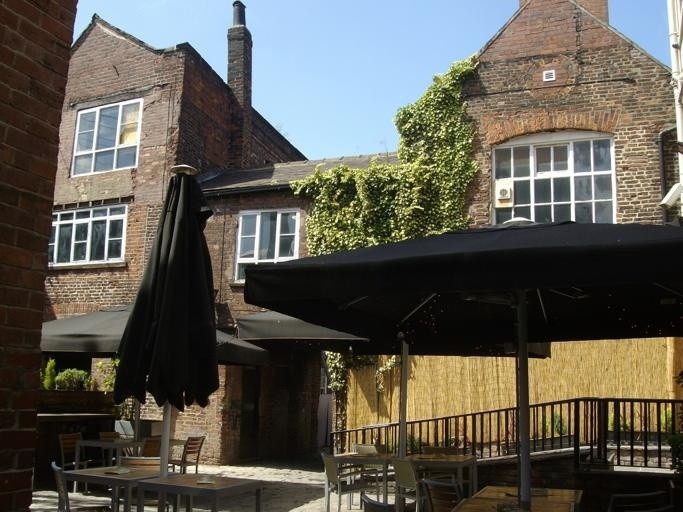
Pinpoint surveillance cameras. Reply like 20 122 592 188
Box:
659 183 683 210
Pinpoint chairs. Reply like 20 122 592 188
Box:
320 443 477 512
52 433 205 512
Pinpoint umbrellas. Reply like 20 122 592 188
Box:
38 303 270 459
240 309 554 461
104 160 223 475
247 216 683 505
